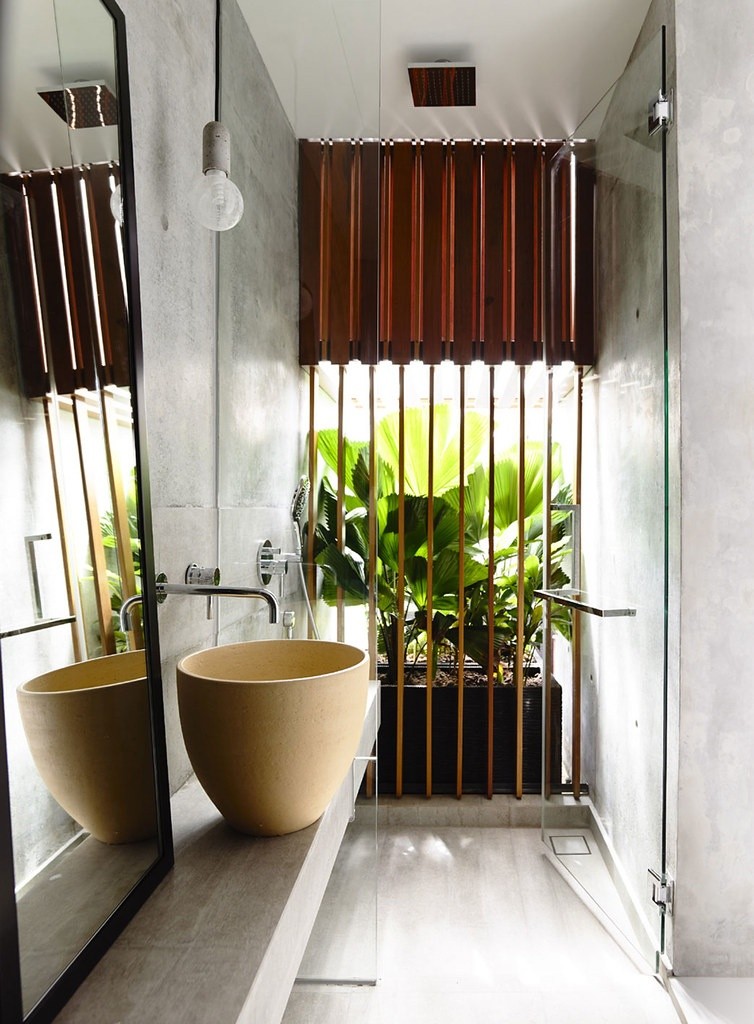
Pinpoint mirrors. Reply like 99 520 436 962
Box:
0 0 174 1024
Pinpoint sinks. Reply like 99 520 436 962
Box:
15 648 160 846
177 638 371 838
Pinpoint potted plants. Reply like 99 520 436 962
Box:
307 402 571 794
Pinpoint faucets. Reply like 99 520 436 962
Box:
120 596 143 633
157 582 280 624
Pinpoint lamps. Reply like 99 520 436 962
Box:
191 0 246 232
